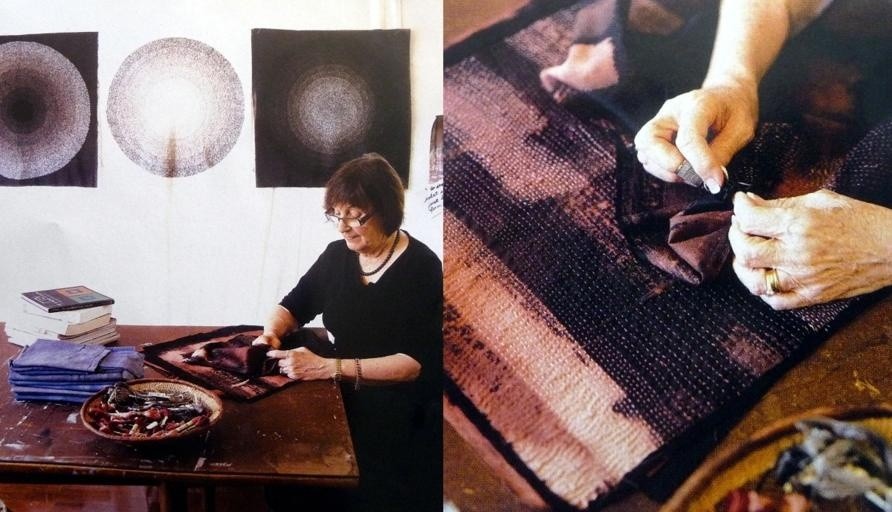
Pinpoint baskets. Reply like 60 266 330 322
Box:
80 377 224 448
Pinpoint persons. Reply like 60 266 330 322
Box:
250 151 443 512
632 0 892 320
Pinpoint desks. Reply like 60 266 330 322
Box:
0 323 362 511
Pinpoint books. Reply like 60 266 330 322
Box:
2 284 122 349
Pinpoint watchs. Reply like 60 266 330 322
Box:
333 357 342 385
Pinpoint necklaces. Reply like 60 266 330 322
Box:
355 229 401 277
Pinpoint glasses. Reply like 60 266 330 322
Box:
325 208 373 228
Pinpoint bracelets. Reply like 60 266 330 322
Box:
350 358 364 394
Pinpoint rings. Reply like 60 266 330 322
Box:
764 270 784 296
279 367 285 376
674 158 703 189
